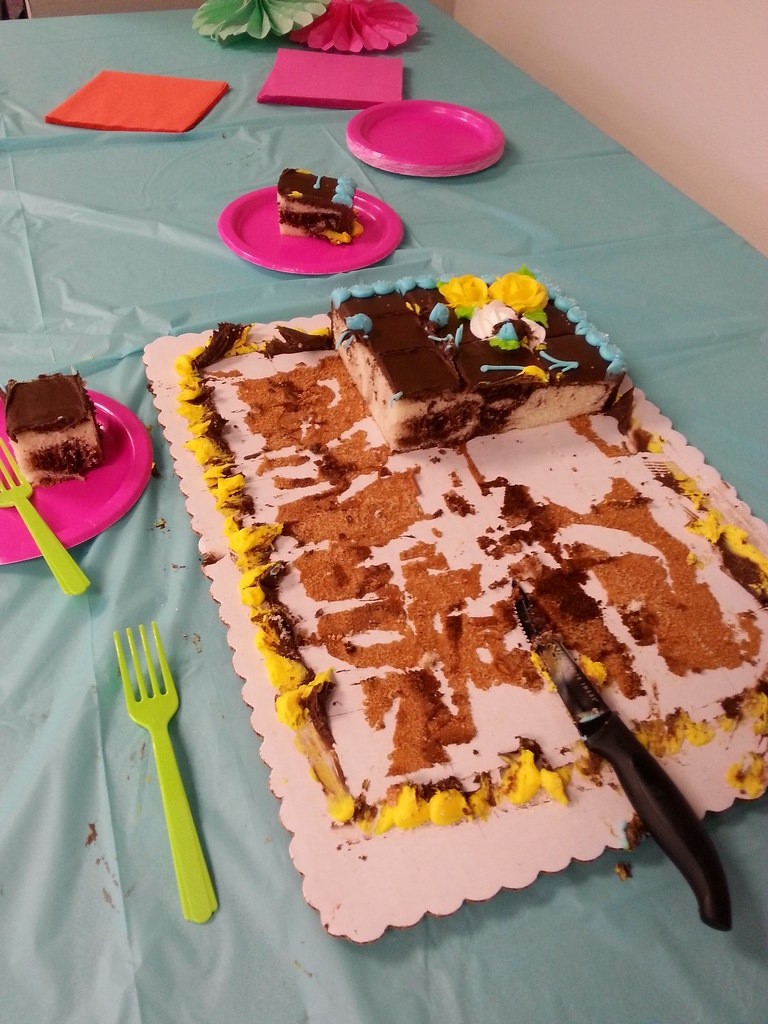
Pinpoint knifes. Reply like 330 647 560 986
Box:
506 578 735 932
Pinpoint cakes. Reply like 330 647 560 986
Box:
5 366 103 487
275 168 357 244
327 264 628 457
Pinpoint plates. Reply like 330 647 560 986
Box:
216 184 406 276
343 99 506 179
0 387 155 568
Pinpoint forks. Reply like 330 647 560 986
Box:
109 619 219 924
0 437 92 597
